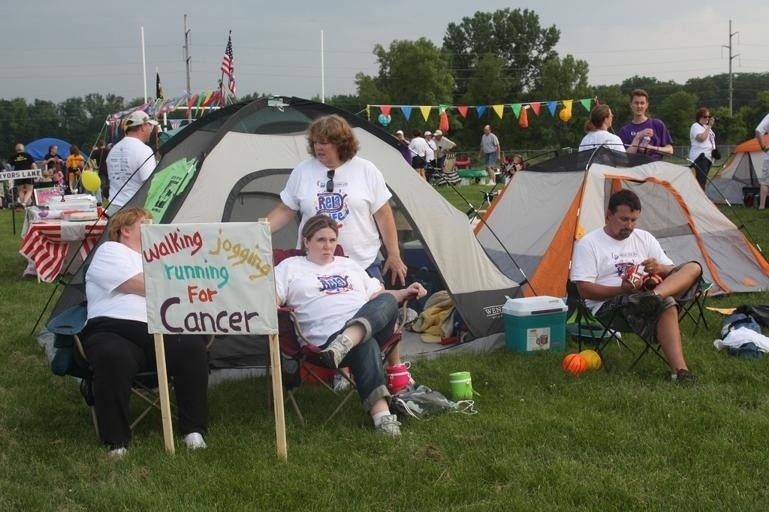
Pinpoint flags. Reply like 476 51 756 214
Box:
218 33 237 94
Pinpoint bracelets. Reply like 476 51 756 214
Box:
762 144 766 150
636 131 640 140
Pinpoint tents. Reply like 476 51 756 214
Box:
30 95 524 384
472 144 769 305
705 132 769 206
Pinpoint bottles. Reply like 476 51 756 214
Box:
449 371 472 400
640 136 651 147
386 364 408 393
97 202 102 220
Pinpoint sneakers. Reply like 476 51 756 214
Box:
674 367 692 388
333 373 353 390
185 432 208 450
318 333 352 368
638 295 661 317
374 414 401 437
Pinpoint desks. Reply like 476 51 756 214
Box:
27 206 109 282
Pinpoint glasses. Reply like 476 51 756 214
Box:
703 116 712 119
327 169 334 192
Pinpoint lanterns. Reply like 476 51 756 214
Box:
559 107 571 122
378 112 391 126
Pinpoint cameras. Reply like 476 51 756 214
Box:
714 117 719 122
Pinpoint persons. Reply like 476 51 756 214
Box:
0 140 112 213
755 113 769 210
258 114 409 392
394 124 527 187
274 214 428 439
106 108 159 218
568 188 703 382
689 107 717 190
576 104 631 170
616 89 674 163
73 208 210 459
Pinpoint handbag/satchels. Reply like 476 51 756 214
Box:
389 380 479 421
712 150 722 159
721 313 763 358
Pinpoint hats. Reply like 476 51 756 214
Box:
424 131 432 136
433 129 443 136
124 109 159 131
395 130 404 135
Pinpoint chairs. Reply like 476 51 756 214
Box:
657 279 714 350
73 334 215 444
278 300 409 427
568 284 674 374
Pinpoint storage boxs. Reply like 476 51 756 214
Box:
500 295 568 353
